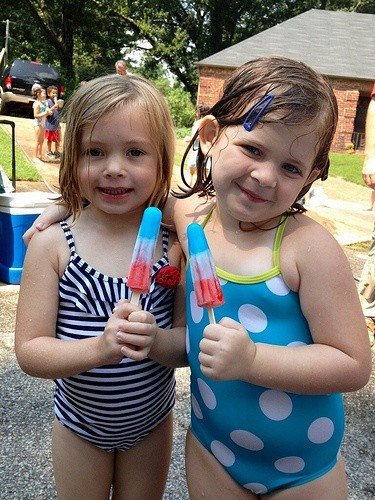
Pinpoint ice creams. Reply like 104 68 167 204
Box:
186 223 225 324
54 96 58 105
45 99 50 112
126 206 162 307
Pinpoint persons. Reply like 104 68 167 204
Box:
46 86 63 157
14 74 189 500
23 56 370 500
356 79 374 319
32 87 53 164
113 60 132 76
186 104 211 187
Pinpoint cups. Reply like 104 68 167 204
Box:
58 100 64 107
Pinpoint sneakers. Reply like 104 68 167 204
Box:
46 152 62 159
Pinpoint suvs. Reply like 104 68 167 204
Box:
0 59 65 115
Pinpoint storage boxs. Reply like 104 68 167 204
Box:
0 192 62 284
